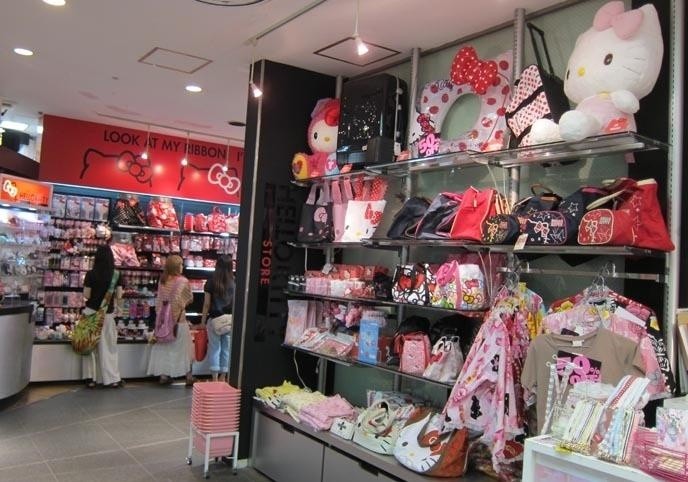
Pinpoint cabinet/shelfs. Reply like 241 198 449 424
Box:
518 430 669 482
250 408 496 482
277 131 672 389
0 203 238 401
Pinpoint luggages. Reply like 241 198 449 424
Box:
338 72 410 175
503 23 568 143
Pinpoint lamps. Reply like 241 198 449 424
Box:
241 1 370 97
94 111 243 171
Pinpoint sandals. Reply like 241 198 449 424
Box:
86 380 96 389
112 379 127 388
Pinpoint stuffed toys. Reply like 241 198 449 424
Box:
287 96 343 181
527 1 665 146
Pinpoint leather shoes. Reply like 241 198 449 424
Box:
186 377 196 386
159 377 167 384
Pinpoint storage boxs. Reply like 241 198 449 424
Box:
190 381 242 458
355 318 397 366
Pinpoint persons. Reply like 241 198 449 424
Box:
200 252 237 382
146 253 207 386
81 243 125 389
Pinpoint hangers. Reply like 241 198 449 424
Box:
572 260 616 332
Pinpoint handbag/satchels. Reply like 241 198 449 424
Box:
296 176 388 242
385 316 430 365
211 313 234 337
391 252 510 313
353 389 416 455
423 335 463 385
387 177 674 253
195 328 208 362
393 406 471 478
397 330 431 377
153 280 183 344
70 306 107 355
